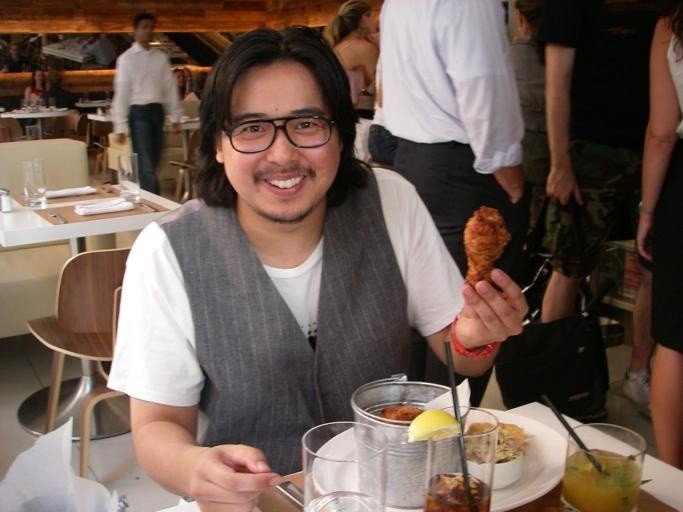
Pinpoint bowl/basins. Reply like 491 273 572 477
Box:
464 452 523 487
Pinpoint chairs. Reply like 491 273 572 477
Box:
1 139 112 339
21 248 136 478
1 94 208 202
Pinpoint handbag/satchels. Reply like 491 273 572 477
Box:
494 309 614 422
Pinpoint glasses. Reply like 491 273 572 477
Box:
220 114 338 154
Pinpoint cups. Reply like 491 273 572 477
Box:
114 154 141 203
562 424 646 509
423 405 495 509
20 160 48 207
300 420 390 512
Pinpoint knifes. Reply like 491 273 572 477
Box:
46 207 64 225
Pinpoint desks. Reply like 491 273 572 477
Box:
0 96 208 478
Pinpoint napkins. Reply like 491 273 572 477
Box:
44 184 96 197
74 198 135 214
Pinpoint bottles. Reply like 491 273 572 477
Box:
0 187 12 214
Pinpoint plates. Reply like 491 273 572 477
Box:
310 410 564 512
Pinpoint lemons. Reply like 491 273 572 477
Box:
406 409 461 444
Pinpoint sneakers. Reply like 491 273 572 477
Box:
620 369 656 422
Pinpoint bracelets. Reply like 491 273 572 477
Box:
638 201 654 215
451 315 497 360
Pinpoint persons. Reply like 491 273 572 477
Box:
106 24 532 512
382 0 529 407
111 13 181 196
540 0 655 423
321 0 379 105
25 69 48 108
172 69 201 101
367 54 397 164
508 0 549 184
636 0 683 470
37 72 74 109
